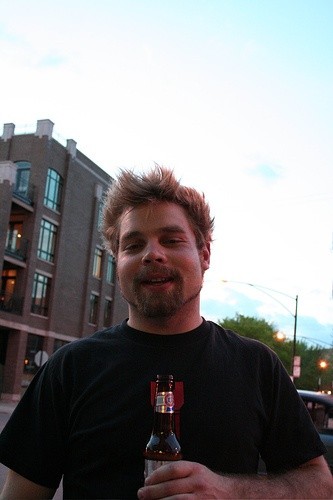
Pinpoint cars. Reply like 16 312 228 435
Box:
297 389 333 475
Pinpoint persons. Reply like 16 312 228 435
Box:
1 162 332 500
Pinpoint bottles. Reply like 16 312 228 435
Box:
144 374 185 482
